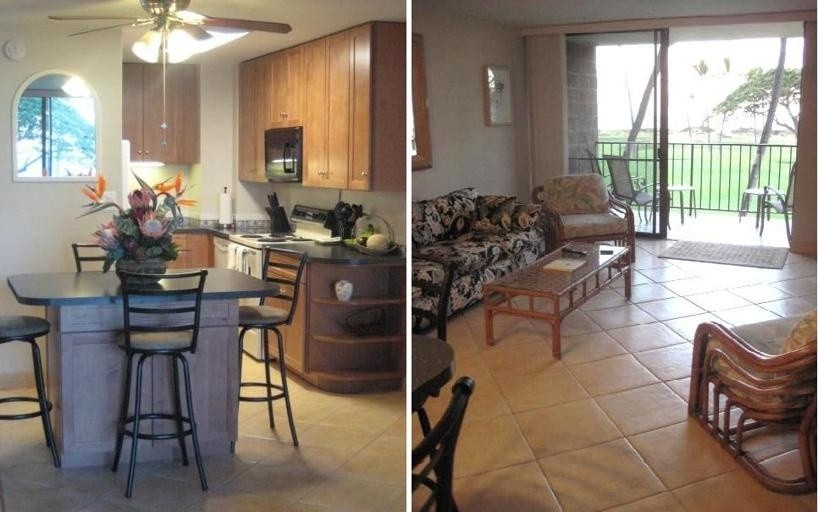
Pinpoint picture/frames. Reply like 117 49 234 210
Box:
483 64 515 127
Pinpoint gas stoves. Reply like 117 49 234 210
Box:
229 232 318 249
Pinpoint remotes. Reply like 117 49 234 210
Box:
561 247 588 255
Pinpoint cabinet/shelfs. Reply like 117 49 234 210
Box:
208 226 266 366
264 239 407 394
268 36 326 194
121 61 201 165
168 215 207 270
238 54 269 185
326 20 406 194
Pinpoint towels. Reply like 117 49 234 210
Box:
235 246 249 274
227 244 236 269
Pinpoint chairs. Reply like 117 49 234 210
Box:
229 250 308 455
411 376 475 512
532 173 636 263
603 154 671 234
685 304 817 494
71 243 120 272
411 252 457 343
759 160 794 247
111 268 210 498
0 315 60 468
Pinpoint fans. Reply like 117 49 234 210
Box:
46 0 292 65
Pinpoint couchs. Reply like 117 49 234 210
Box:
408 186 568 333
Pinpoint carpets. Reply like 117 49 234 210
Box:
656 239 790 270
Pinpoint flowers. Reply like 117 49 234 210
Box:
70 168 200 274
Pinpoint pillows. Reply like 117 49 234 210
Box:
470 195 516 234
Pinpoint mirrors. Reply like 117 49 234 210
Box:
10 68 102 184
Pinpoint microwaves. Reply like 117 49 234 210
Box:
264 126 303 184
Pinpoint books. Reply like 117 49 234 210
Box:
544 258 589 273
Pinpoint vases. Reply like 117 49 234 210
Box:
115 257 168 286
